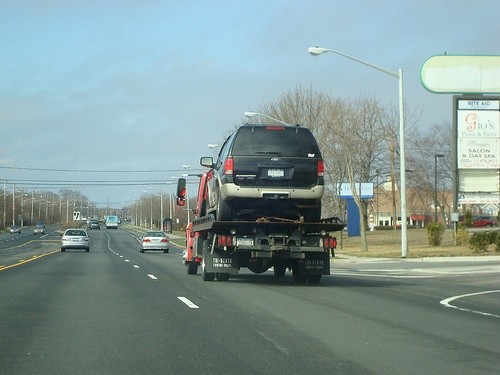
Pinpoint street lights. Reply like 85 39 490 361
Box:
434 154 445 222
0 187 95 235
119 143 223 231
244 112 292 127
308 47 408 257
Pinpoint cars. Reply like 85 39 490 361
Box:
61 228 90 252
10 225 22 234
34 223 46 235
140 231 169 252
469 216 497 227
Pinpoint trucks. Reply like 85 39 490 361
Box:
177 172 347 283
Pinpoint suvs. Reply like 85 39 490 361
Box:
200 123 325 222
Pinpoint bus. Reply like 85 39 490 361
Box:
73 211 127 230
